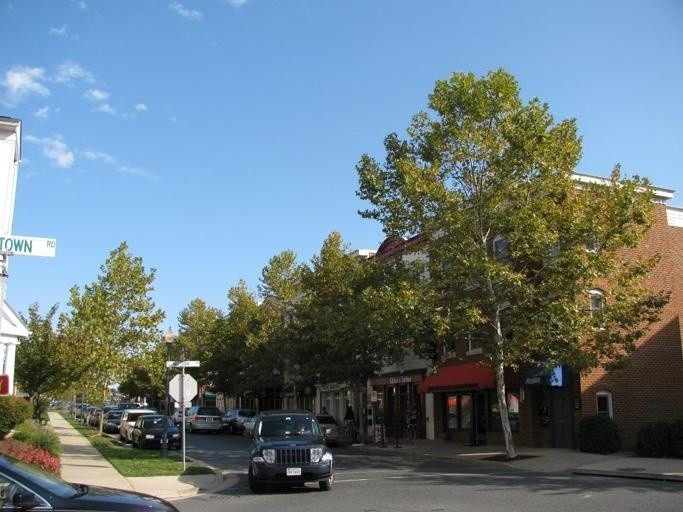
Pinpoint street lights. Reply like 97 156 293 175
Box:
162 330 173 448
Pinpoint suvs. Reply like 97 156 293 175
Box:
173 406 258 434
248 411 332 490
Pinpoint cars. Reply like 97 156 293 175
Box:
53 401 183 451
0 452 177 512
317 414 338 441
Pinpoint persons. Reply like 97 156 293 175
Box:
342 406 355 424
322 406 330 414
406 400 419 440
